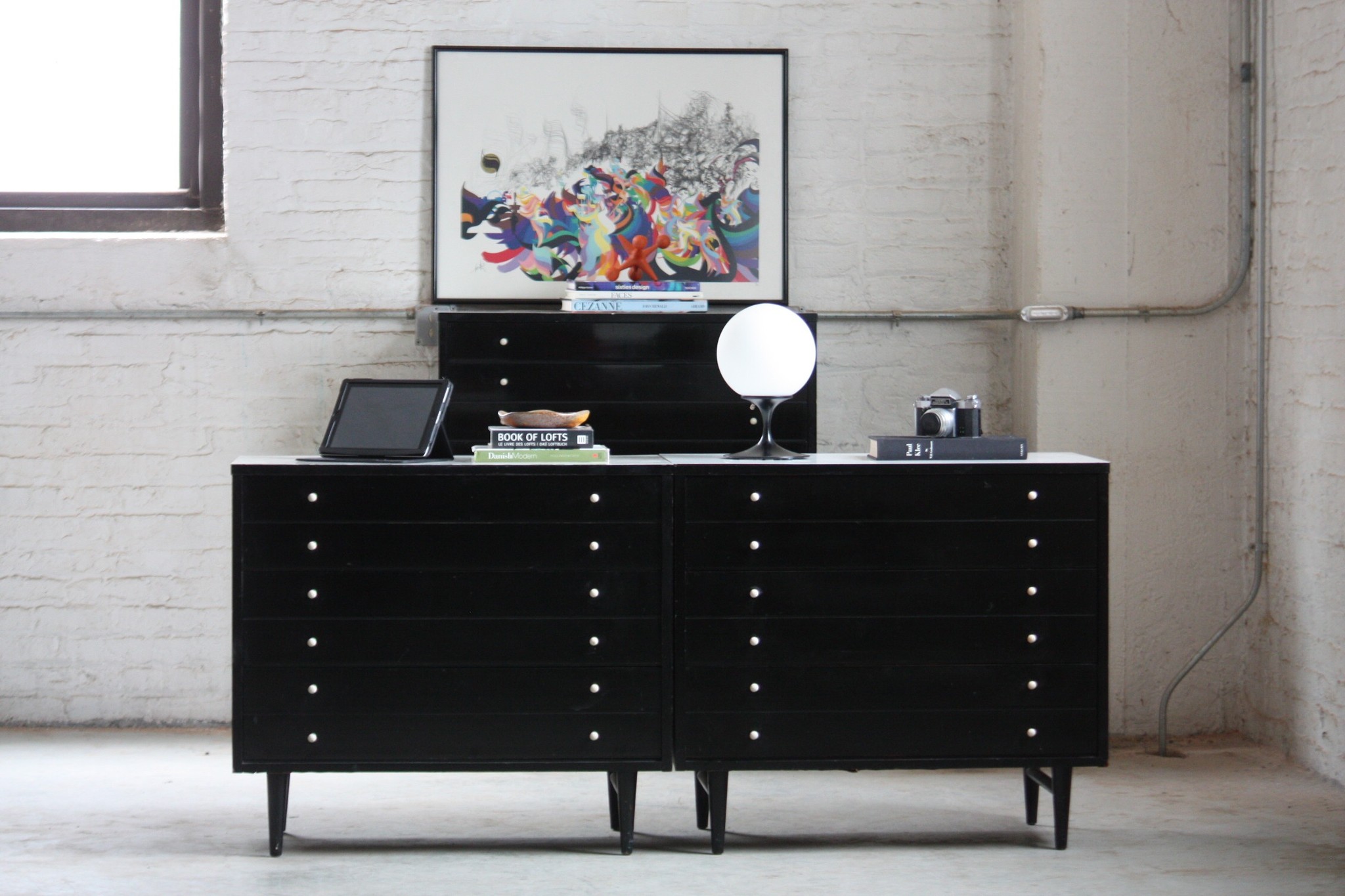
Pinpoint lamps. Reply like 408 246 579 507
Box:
717 304 817 460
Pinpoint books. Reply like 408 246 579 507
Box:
472 425 611 465
867 434 1027 460
560 279 708 313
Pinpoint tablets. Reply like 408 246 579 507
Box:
318 377 455 459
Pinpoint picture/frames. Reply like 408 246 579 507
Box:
430 46 789 304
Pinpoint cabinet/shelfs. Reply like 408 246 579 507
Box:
229 310 1111 855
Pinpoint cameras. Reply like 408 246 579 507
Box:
911 384 982 439
